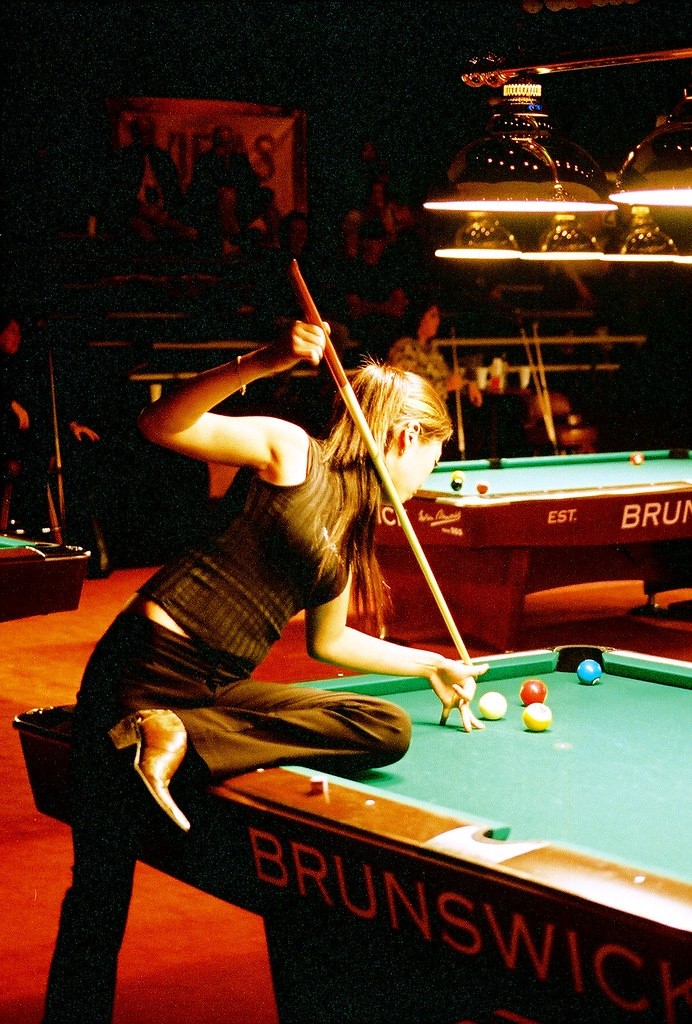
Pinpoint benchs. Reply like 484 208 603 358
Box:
59 230 649 403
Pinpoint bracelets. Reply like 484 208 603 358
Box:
237 356 246 395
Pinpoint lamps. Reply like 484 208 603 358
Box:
522 214 604 260
604 206 680 262
610 88 692 207
434 211 522 259
423 76 619 212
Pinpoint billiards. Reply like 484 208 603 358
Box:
478 689 507 720
477 482 488 494
451 470 464 492
520 680 547 706
577 660 602 685
523 703 551 732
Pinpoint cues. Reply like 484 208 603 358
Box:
521 323 560 454
289 259 479 682
449 326 467 460
40 344 69 544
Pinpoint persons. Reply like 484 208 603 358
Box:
40 321 453 1024
104 116 526 458
0 315 100 542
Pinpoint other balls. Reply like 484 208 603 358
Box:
631 450 644 465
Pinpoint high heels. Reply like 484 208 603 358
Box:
107 709 191 832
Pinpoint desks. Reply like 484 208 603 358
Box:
374 446 692 653
0 533 90 622
15 640 692 1024
